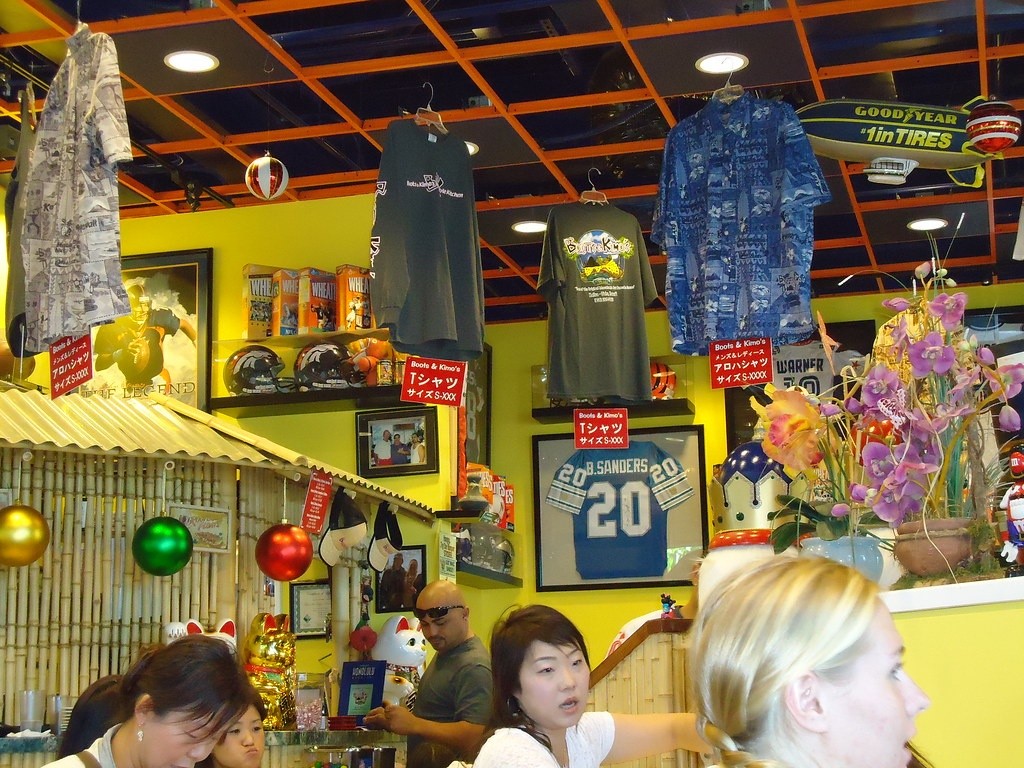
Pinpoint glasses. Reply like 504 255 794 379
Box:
412 605 464 619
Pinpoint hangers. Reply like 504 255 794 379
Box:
415 82 449 134
711 57 745 103
25 82 38 126
579 168 609 207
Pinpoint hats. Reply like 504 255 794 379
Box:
368 501 403 572
317 487 367 567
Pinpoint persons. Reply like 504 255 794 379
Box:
363 580 493 768
380 553 422 608
310 301 336 327
94 284 197 393
251 303 272 321
281 303 299 326
688 554 930 768
474 605 711 768
373 430 426 465
41 634 251 768
195 685 267 768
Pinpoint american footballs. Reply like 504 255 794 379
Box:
123 338 150 379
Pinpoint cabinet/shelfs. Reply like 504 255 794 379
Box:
210 325 418 409
455 510 523 587
529 356 695 416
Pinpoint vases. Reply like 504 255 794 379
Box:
798 535 883 586
895 517 977 575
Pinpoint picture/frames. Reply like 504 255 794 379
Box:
375 545 428 613
355 405 439 480
724 320 877 457
65 246 214 413
531 423 708 592
288 578 332 640
949 304 1024 474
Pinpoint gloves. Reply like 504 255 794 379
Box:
113 338 144 364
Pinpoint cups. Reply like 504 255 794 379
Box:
19 689 46 733
47 696 72 734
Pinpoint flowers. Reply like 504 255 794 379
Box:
751 210 1024 555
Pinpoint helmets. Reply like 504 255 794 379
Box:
125 284 153 324
223 345 285 395
292 340 356 393
